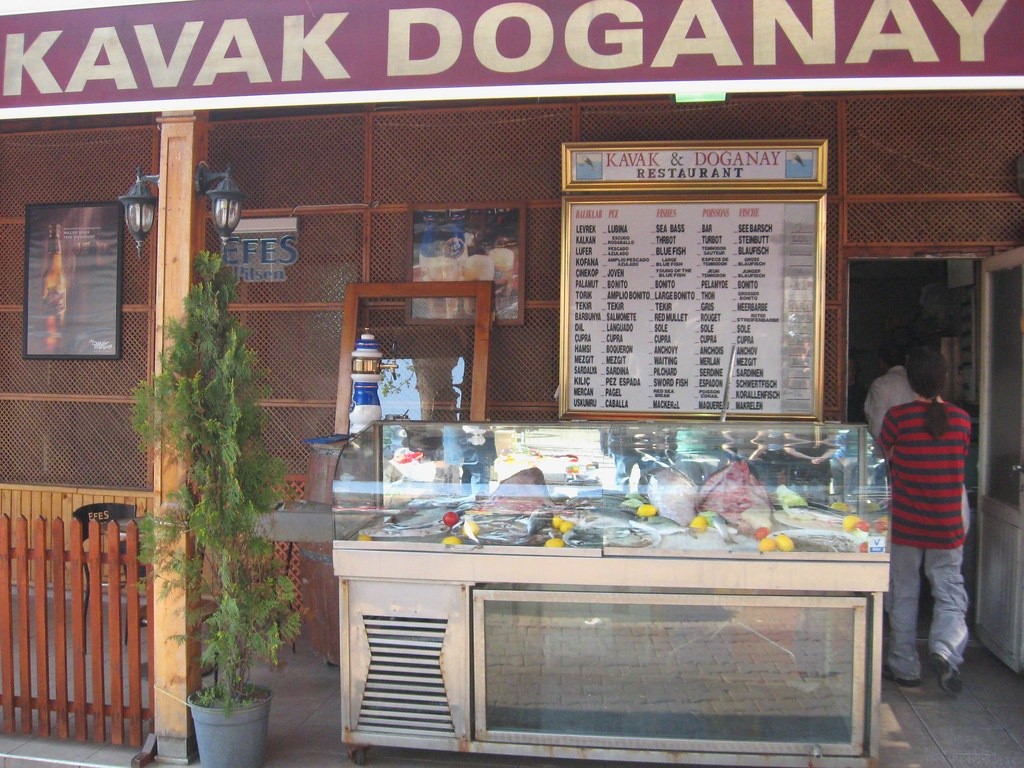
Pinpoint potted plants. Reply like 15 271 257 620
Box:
126 242 303 767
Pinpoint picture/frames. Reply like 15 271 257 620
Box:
21 201 124 360
402 201 529 326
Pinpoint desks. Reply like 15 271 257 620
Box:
101 515 152 545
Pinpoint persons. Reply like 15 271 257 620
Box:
864 342 916 615
847 354 868 423
878 348 972 693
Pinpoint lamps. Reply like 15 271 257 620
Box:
197 158 249 247
117 167 159 260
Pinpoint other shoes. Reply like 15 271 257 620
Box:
881 664 923 687
926 653 963 694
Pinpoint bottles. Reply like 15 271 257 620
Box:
439 209 468 262
419 212 442 274
41 222 67 333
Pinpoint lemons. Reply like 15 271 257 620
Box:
638 506 657 515
545 518 574 548
759 534 793 552
688 516 706 532
844 514 861 531
443 522 480 545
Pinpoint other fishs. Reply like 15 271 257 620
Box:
333 460 773 544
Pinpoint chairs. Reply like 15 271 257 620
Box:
130 534 220 689
68 502 153 655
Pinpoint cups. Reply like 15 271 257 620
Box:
462 254 494 317
423 255 458 318
488 247 514 311
